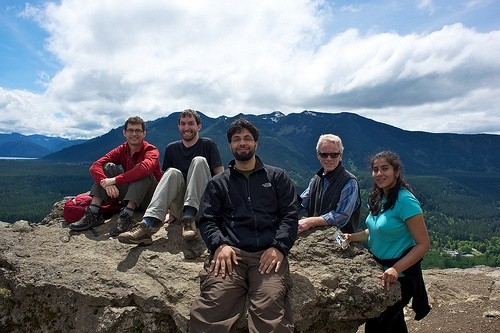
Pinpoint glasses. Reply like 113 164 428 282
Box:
125 129 143 134
319 153 340 158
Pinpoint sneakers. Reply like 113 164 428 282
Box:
108 211 132 237
118 222 152 246
69 212 104 232
181 216 198 241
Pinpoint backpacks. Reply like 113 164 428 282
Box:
64 194 112 223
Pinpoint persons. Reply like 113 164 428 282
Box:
190 118 303 333
340 151 430 333
118 108 224 246
297 134 361 234
69 116 163 236
